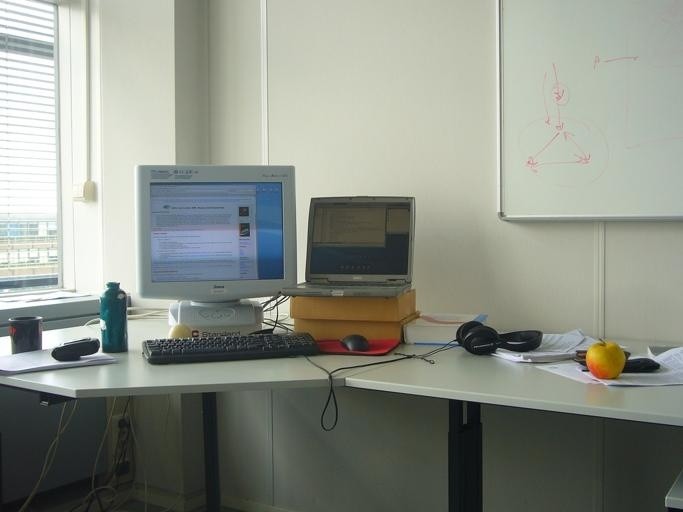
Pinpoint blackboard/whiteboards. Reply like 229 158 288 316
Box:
498 0 683 222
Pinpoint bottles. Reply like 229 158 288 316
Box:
99 281 128 353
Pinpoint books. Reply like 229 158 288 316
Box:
489 333 577 363
403 313 490 347
647 344 682 359
0 345 118 377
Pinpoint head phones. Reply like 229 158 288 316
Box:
456 320 543 355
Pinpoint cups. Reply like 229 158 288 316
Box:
7 314 42 355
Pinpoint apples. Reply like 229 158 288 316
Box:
585 337 626 379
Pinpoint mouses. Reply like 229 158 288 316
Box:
341 335 369 352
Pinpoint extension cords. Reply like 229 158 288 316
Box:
110 415 131 475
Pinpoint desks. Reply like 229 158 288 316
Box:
0 310 683 512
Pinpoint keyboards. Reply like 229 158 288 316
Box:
141 331 319 364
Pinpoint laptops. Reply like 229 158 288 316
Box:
281 196 416 297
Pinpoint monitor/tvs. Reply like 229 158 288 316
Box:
135 164 297 328
